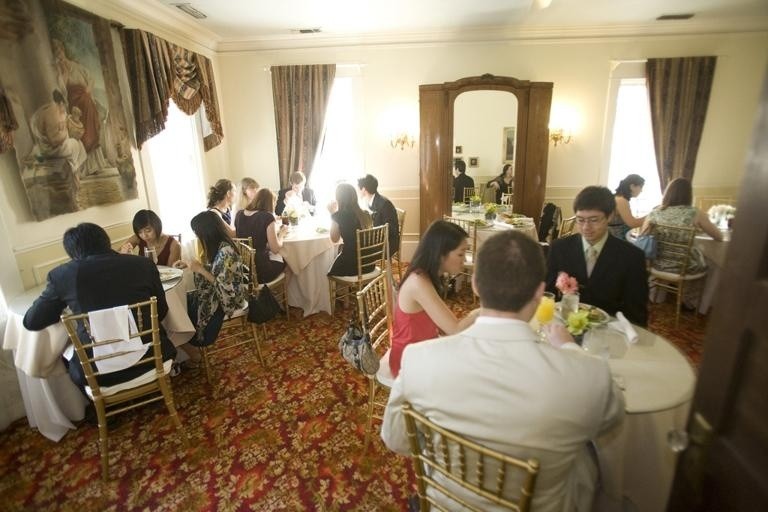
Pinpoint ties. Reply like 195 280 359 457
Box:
585 247 598 279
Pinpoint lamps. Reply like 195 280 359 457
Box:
550 129 571 146
391 135 414 150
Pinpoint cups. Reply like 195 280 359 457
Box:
561 294 580 329
281 217 290 230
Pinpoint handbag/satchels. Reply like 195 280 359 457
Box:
338 295 380 374
247 252 281 325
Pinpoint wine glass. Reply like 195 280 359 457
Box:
144 247 160 273
536 293 555 339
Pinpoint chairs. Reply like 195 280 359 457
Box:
357 271 399 454
403 401 538 512
231 236 289 321
464 186 480 204
61 296 191 481
502 193 513 205
646 223 708 324
329 223 388 320
441 214 479 303
183 231 265 383
540 215 576 246
169 234 182 242
385 209 406 284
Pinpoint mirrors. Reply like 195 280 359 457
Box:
450 90 518 205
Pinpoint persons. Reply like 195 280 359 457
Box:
116 209 182 268
50 38 102 175
237 177 259 210
389 220 481 378
30 89 88 184
23 223 181 429
380 231 627 512
487 164 514 205
358 174 400 260
607 174 647 241
207 179 237 239
275 172 316 218
642 179 723 313
173 211 251 378
327 183 376 296
235 188 291 315
453 160 474 204
546 186 649 330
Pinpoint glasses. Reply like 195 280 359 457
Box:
576 215 608 224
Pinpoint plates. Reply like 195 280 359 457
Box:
560 303 612 327
155 265 184 281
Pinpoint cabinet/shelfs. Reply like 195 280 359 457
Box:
419 74 553 243
